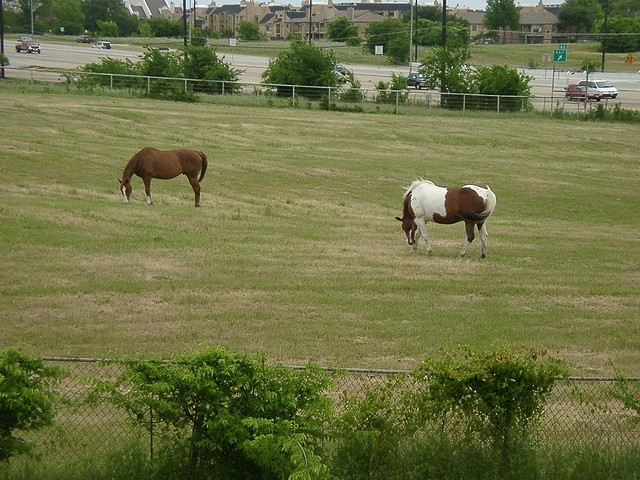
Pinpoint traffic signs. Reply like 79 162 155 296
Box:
375 46 383 54
542 53 551 61
554 50 566 62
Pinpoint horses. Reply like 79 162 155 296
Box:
118 147 208 208
395 177 497 262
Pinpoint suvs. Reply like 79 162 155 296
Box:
406 73 437 90
92 41 112 52
577 80 618 98
76 35 91 44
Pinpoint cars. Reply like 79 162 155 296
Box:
16 37 41 54
565 85 603 101
